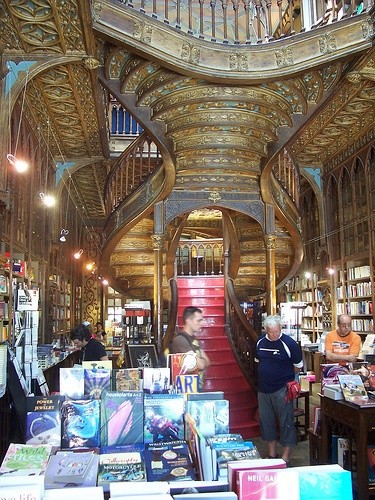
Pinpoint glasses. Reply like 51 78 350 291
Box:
339 324 351 328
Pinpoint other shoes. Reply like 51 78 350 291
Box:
263 454 278 460
283 459 289 467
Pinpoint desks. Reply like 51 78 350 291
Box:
308 392 375 500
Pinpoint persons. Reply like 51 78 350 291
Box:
251 315 304 469
93 322 108 346
170 305 211 371
69 325 110 364
87 324 96 340
324 313 363 365
144 408 179 444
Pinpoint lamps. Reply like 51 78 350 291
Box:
6 68 83 261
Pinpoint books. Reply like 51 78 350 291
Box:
284 270 324 345
368 390 375 401
0 250 71 372
0 360 368 500
110 306 155 346
368 444 375 489
334 264 375 359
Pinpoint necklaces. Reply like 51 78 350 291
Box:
268 339 279 355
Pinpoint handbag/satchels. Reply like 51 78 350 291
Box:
288 381 301 400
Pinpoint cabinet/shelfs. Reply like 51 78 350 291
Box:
0 231 83 469
293 389 309 441
104 287 169 371
239 249 375 382
